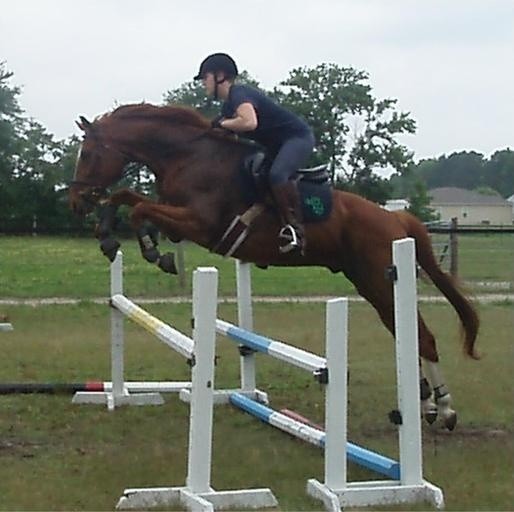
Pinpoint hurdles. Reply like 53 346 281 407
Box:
179 236 446 510
70 251 277 512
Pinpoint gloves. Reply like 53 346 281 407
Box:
211 115 226 129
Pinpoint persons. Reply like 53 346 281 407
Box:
194 51 317 251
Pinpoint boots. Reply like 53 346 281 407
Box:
270 180 307 258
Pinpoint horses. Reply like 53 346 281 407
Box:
68 102 484 431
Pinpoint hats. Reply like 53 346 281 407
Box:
194 54 239 81
